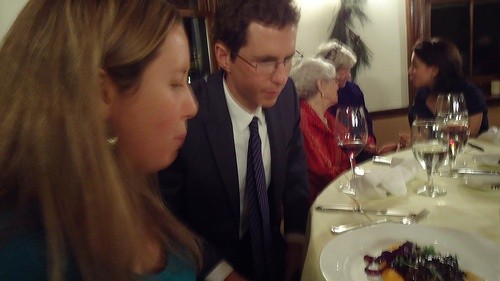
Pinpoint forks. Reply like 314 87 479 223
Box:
330 208 430 233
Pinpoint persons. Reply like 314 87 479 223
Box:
158 0 312 281
291 57 356 199
314 40 376 164
0 0 203 281
379 37 489 155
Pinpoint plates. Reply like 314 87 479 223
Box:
319 223 500 281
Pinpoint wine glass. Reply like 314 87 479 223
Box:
335 106 369 195
411 120 450 198
432 92 469 179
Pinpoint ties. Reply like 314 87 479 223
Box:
245 116 274 281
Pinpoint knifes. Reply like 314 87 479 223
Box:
315 204 411 216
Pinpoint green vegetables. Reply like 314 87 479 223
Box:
392 242 463 281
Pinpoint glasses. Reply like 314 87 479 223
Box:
326 42 343 60
225 47 303 74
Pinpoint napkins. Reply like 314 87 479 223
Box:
350 156 418 200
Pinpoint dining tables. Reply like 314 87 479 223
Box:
302 138 500 281
